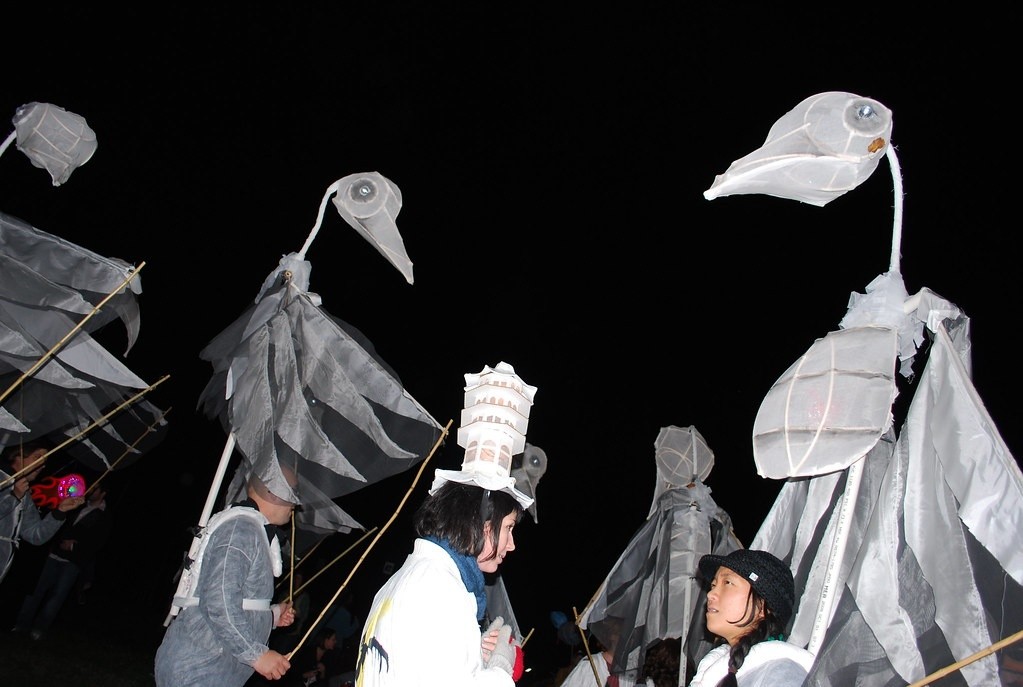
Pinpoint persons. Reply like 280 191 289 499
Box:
152 458 296 687
0 440 86 582
560 616 656 687
636 638 697 687
290 628 338 687
688 547 832 687
351 455 535 687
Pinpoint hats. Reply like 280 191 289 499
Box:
696 549 795 632
